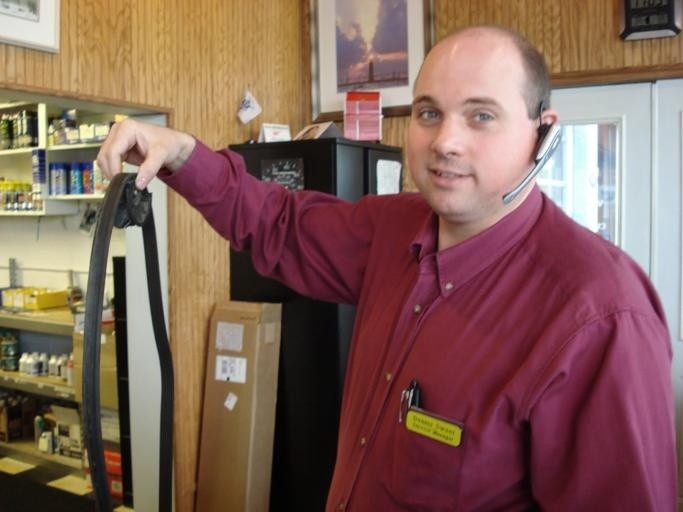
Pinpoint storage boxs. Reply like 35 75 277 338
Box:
194 297 281 510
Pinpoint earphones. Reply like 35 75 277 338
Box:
534 101 563 165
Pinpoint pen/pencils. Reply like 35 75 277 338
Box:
398 379 421 424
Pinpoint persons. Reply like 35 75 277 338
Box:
95 23 680 511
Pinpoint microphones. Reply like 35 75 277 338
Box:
502 166 543 205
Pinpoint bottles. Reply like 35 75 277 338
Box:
17 348 73 387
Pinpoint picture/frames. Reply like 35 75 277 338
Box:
296 1 433 125
0 0 66 58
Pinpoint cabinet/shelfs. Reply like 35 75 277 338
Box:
2 299 119 478
2 103 116 216
